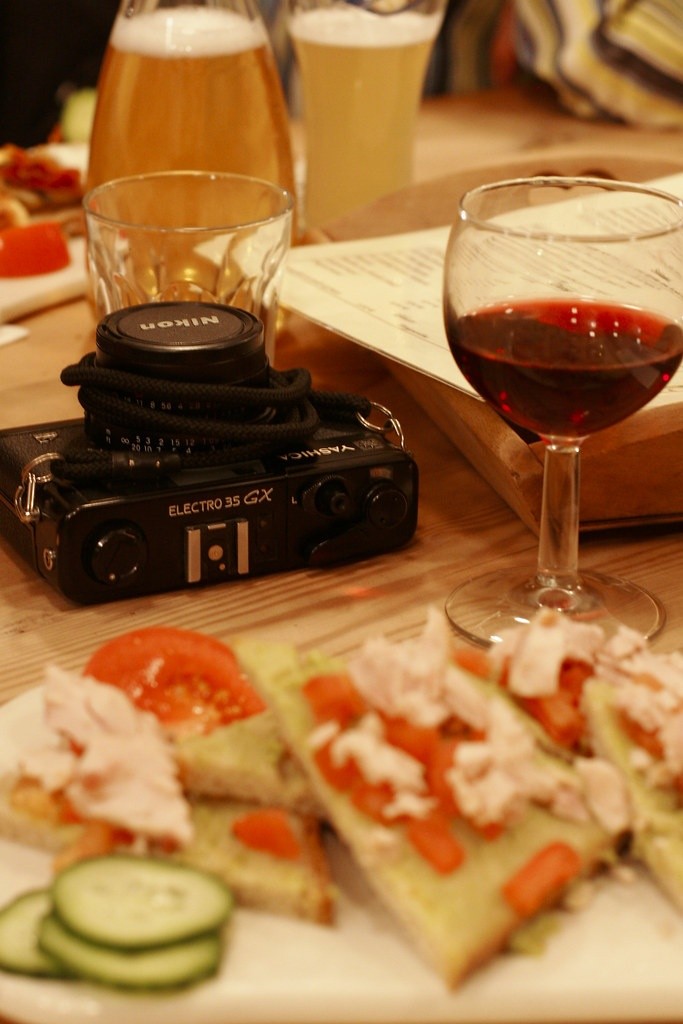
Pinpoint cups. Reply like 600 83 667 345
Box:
287 0 447 225
83 172 297 367
82 0 296 266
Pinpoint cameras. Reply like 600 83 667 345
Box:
0 299 418 604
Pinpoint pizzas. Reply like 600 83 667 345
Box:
0 626 342 926
257 607 683 991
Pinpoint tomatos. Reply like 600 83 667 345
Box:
0 221 70 277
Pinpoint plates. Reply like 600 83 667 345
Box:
0 682 683 1024
0 138 91 324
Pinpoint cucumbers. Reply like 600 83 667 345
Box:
0 853 233 991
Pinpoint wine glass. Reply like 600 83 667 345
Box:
442 175 683 649
